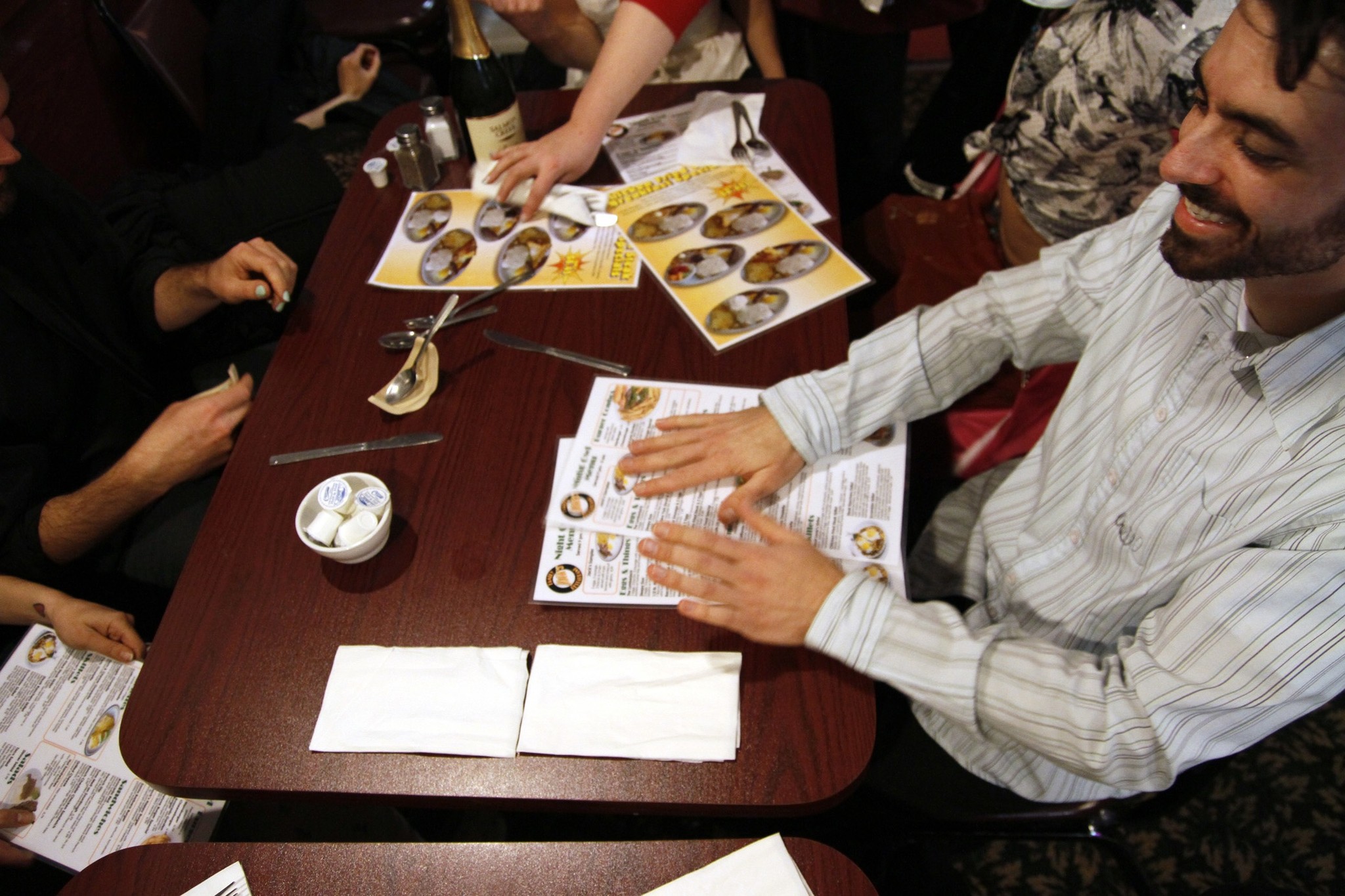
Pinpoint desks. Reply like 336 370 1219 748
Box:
63 80 882 896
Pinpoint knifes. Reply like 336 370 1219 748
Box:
269 431 443 466
484 329 632 377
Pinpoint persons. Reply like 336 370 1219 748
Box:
617 1 1345 896
470 0 785 221
0 0 419 896
906 0 1238 266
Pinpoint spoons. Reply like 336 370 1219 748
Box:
379 306 498 403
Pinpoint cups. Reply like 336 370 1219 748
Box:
295 472 392 564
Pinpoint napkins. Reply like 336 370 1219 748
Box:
676 90 766 167
309 646 530 758
516 644 742 763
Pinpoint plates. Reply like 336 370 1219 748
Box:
84 704 120 756
422 227 477 286
860 560 889 588
403 193 452 243
548 212 589 244
5 768 43 814
594 533 623 560
705 287 789 333
627 202 706 241
475 198 522 244
498 227 552 287
664 242 746 286
612 454 639 494
740 241 830 284
851 521 886 559
27 632 57 664
700 200 786 239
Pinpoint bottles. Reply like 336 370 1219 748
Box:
394 124 441 190
448 0 527 168
418 96 460 165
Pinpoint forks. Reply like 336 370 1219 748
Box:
403 270 509 329
731 101 770 158
